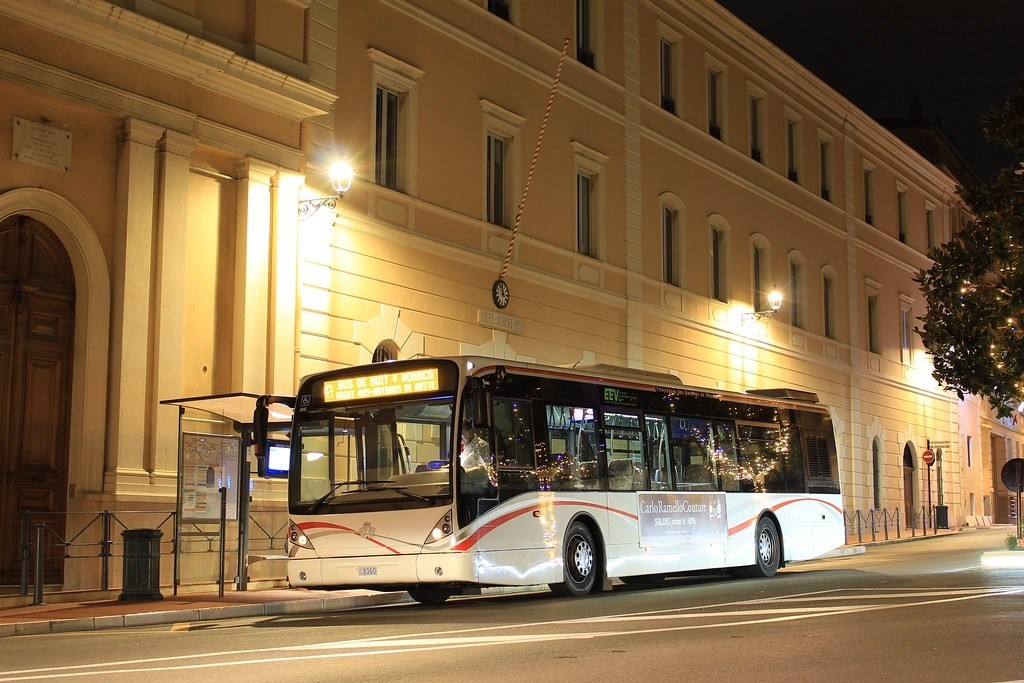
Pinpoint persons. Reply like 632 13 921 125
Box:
459 419 490 488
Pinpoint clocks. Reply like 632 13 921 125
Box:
492 280 510 310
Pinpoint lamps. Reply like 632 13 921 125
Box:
298 160 355 221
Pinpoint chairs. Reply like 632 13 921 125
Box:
549 452 786 490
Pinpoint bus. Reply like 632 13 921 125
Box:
254 355 845 605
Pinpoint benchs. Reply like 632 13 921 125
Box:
247 553 289 568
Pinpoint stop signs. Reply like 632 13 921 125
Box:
922 450 934 464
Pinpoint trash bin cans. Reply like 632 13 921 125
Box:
118 528 164 600
935 505 949 529
